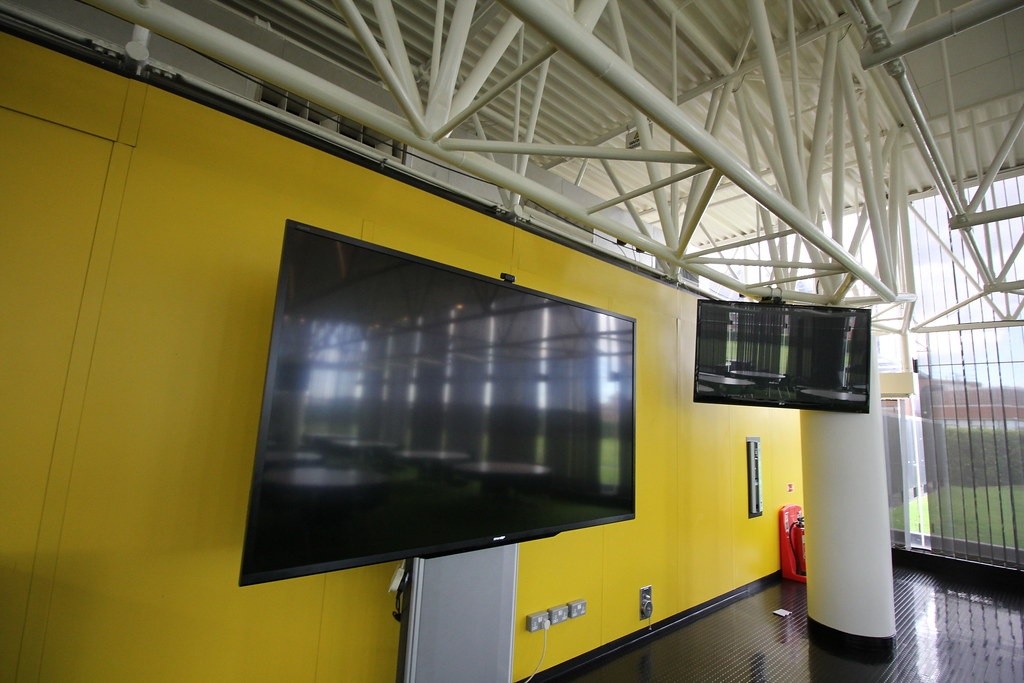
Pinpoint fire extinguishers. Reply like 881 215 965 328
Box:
789 516 806 576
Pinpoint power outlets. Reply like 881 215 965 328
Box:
525 612 550 633
547 605 569 625
566 600 587 618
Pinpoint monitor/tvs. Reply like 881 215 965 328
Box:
693 300 872 414
238 218 636 587
